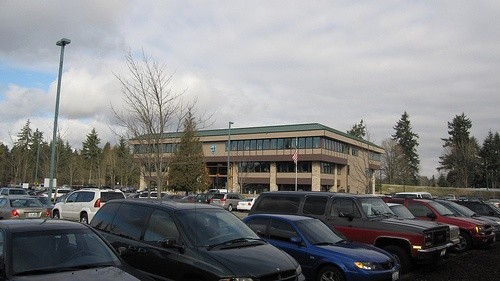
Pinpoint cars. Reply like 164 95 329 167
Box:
0 218 155 281
0 182 219 204
0 195 50 221
225 213 403 281
237 198 256 212
362 190 500 253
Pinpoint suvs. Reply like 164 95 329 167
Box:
248 191 453 275
83 198 307 281
51 186 126 224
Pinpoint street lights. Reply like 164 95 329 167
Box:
226 121 234 195
43 37 72 211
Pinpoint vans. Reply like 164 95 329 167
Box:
210 192 245 213
